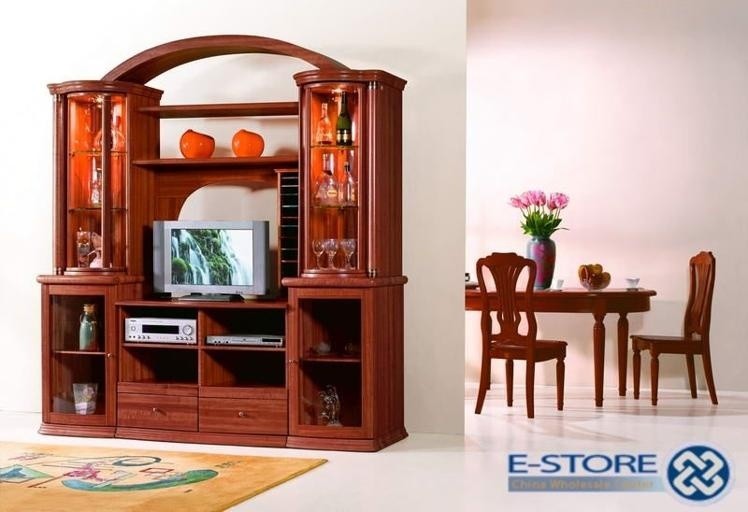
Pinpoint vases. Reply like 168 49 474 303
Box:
524 233 557 289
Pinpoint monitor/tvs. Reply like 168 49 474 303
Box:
152 220 271 300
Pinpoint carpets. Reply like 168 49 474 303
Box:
0 436 327 512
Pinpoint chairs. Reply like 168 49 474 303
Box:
630 250 720 405
475 251 568 418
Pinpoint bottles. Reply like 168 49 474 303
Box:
312 150 356 207
79 303 98 352
316 104 333 144
94 113 126 152
335 92 353 146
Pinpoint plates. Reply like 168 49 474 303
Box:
465 281 480 289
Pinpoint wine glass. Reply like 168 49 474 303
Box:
311 237 356 271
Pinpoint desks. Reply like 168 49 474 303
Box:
465 282 657 407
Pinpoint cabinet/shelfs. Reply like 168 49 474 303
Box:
115 296 290 447
130 101 299 174
275 166 303 296
36 271 153 439
281 276 411 452
45 80 165 278
292 67 408 278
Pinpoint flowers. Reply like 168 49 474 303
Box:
507 187 570 242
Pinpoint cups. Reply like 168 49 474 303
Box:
552 277 563 289
627 278 640 289
90 170 102 208
72 382 98 415
76 232 91 268
